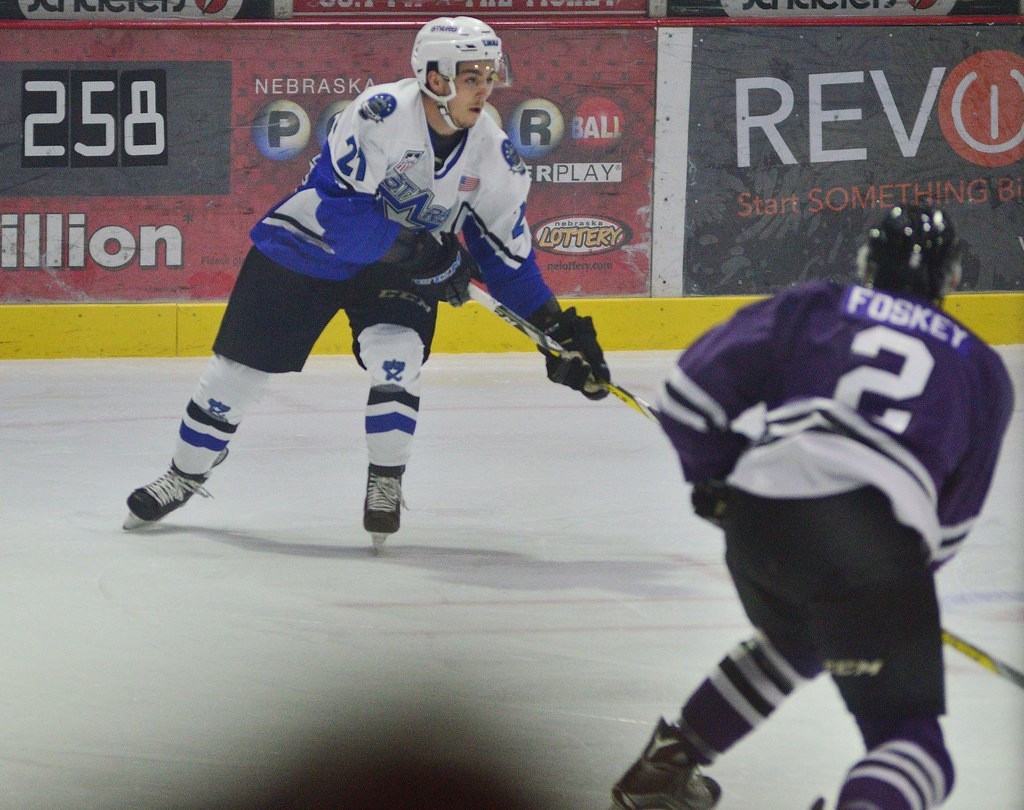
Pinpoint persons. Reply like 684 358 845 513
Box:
124 15 614 557
600 198 1017 810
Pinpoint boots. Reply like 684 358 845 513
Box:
612 719 721 809
122 445 231 532
363 460 408 554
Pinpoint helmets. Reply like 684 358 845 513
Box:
410 15 504 86
867 203 956 296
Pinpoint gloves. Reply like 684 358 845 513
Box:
539 310 610 400
401 226 481 306
